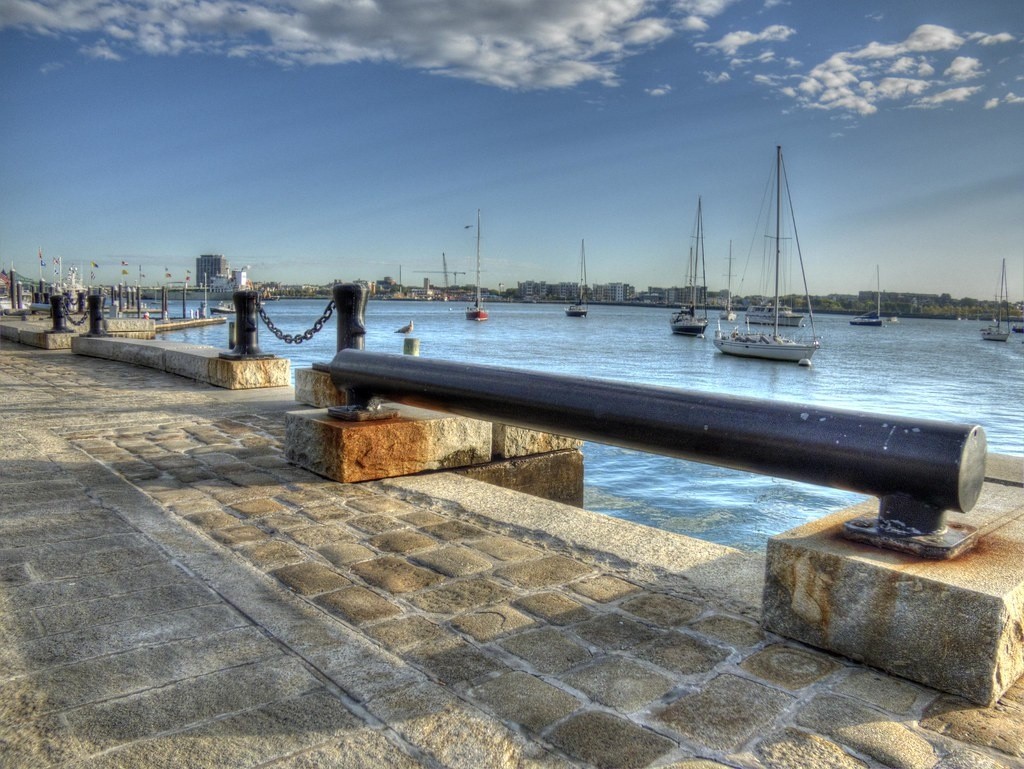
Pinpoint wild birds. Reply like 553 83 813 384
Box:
394 320 414 339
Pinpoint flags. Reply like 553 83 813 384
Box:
165 273 171 278
92 272 96 279
122 260 128 265
53 258 60 264
91 261 98 267
186 276 190 280
39 249 42 258
41 260 45 265
122 269 128 273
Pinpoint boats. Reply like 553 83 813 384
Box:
209 302 236 314
744 304 805 327
1012 324 1024 334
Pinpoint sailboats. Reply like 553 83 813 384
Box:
563 239 590 317
711 142 823 362
981 257 1012 342
849 264 883 327
465 208 490 321
718 237 737 321
667 194 710 337
